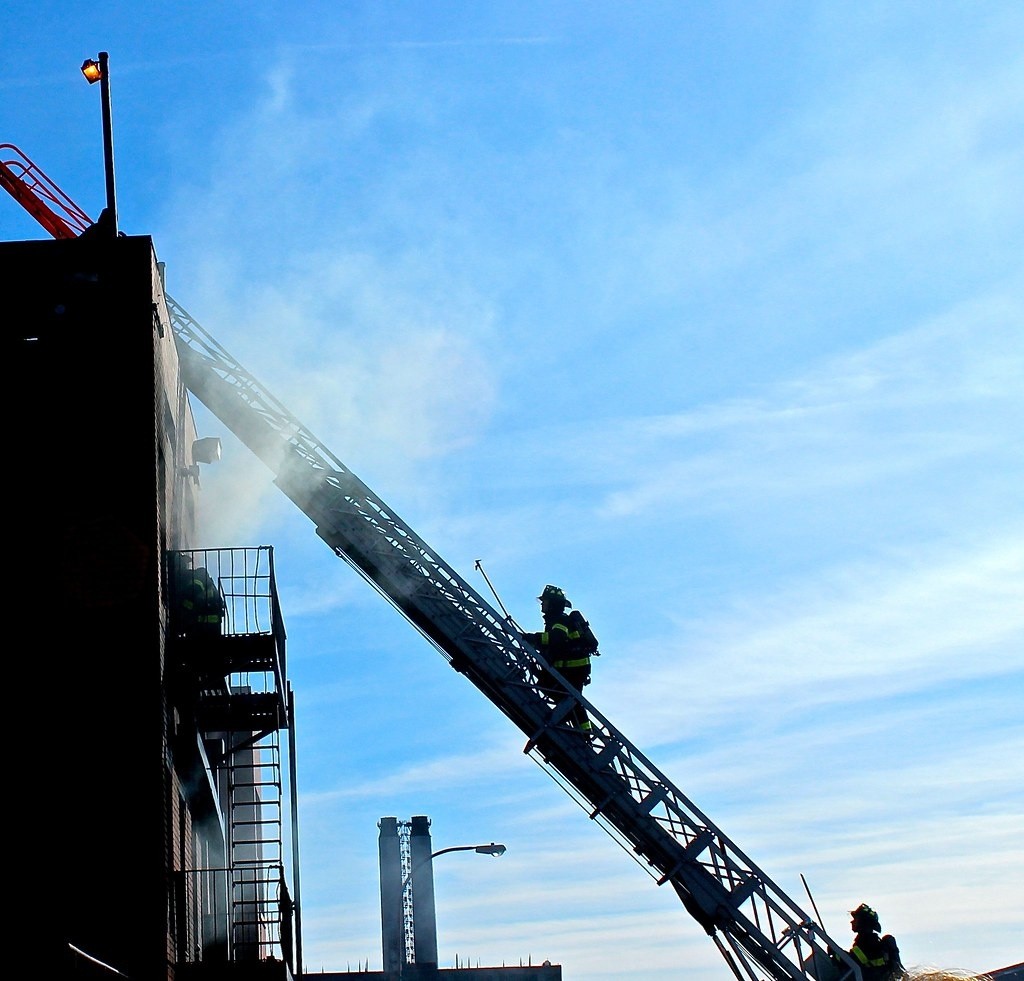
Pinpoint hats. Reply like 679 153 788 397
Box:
848 903 882 933
535 585 572 609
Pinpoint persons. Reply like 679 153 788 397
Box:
515 586 591 734
175 552 221 637
827 904 890 981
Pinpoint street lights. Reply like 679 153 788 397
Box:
80 52 119 236
399 843 507 981
